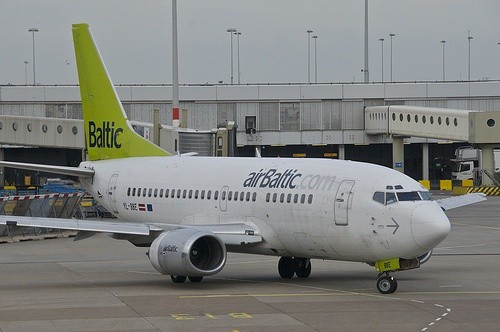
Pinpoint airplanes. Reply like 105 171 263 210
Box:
0 22 487 293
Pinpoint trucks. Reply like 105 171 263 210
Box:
450 148 500 179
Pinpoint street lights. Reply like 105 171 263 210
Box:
24 28 39 85
379 38 384 83
307 30 318 83
441 41 446 81
227 29 242 85
468 37 473 81
388 34 395 82
